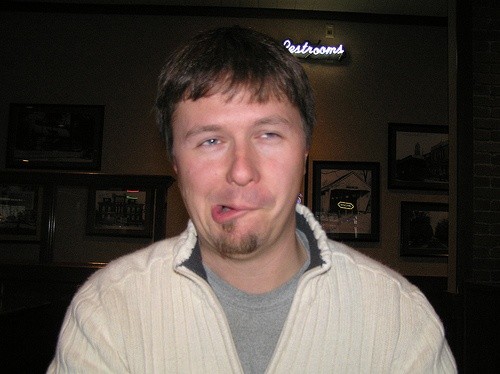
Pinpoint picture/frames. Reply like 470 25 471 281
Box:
401 201 450 259
5 102 106 172
0 180 50 244
312 159 381 243
86 186 155 238
388 122 450 193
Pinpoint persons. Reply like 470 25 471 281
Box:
45 28 457 374
